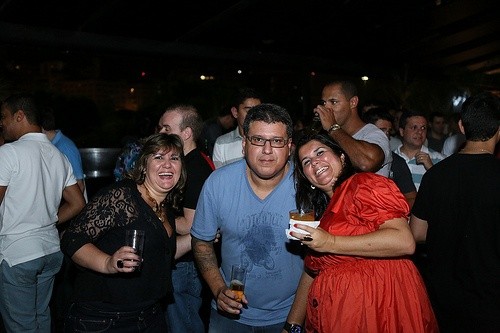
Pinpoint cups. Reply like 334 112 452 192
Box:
230 263 246 302
125 230 145 266
311 111 322 133
289 208 314 238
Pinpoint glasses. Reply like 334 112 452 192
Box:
245 135 289 147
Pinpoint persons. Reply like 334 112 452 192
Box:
0 93 88 333
190 104 307 333
290 130 417 333
159 78 467 333
410 91 500 333
48 132 220 333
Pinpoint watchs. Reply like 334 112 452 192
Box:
329 124 341 134
284 321 304 333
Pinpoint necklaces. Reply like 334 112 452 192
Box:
143 183 167 222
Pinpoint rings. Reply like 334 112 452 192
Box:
116 259 125 268
304 234 313 241
420 157 423 160
